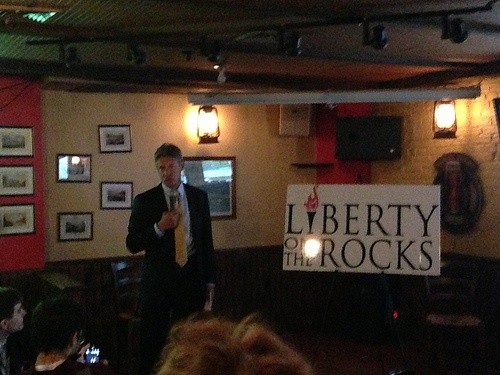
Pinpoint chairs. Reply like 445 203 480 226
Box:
114 268 140 368
424 275 485 372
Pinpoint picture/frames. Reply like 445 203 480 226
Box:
98 124 132 153
100 182 133 209
0 203 36 236
181 157 237 219
0 164 35 196
57 212 94 242
0 126 34 158
57 154 92 184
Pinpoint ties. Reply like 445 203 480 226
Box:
174 192 187 266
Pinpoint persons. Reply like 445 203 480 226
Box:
157 312 315 375
15 301 115 375
0 291 25 375
126 143 216 375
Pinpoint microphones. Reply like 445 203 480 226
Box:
170 195 176 212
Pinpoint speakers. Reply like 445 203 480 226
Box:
335 115 402 159
278 103 311 136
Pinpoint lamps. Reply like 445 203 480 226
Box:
362 21 389 49
58 44 82 67
441 14 468 44
197 105 220 144
200 40 221 63
276 29 302 57
126 41 147 65
431 100 458 139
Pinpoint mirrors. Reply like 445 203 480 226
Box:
433 153 484 235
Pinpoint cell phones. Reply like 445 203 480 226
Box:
85 346 101 367
